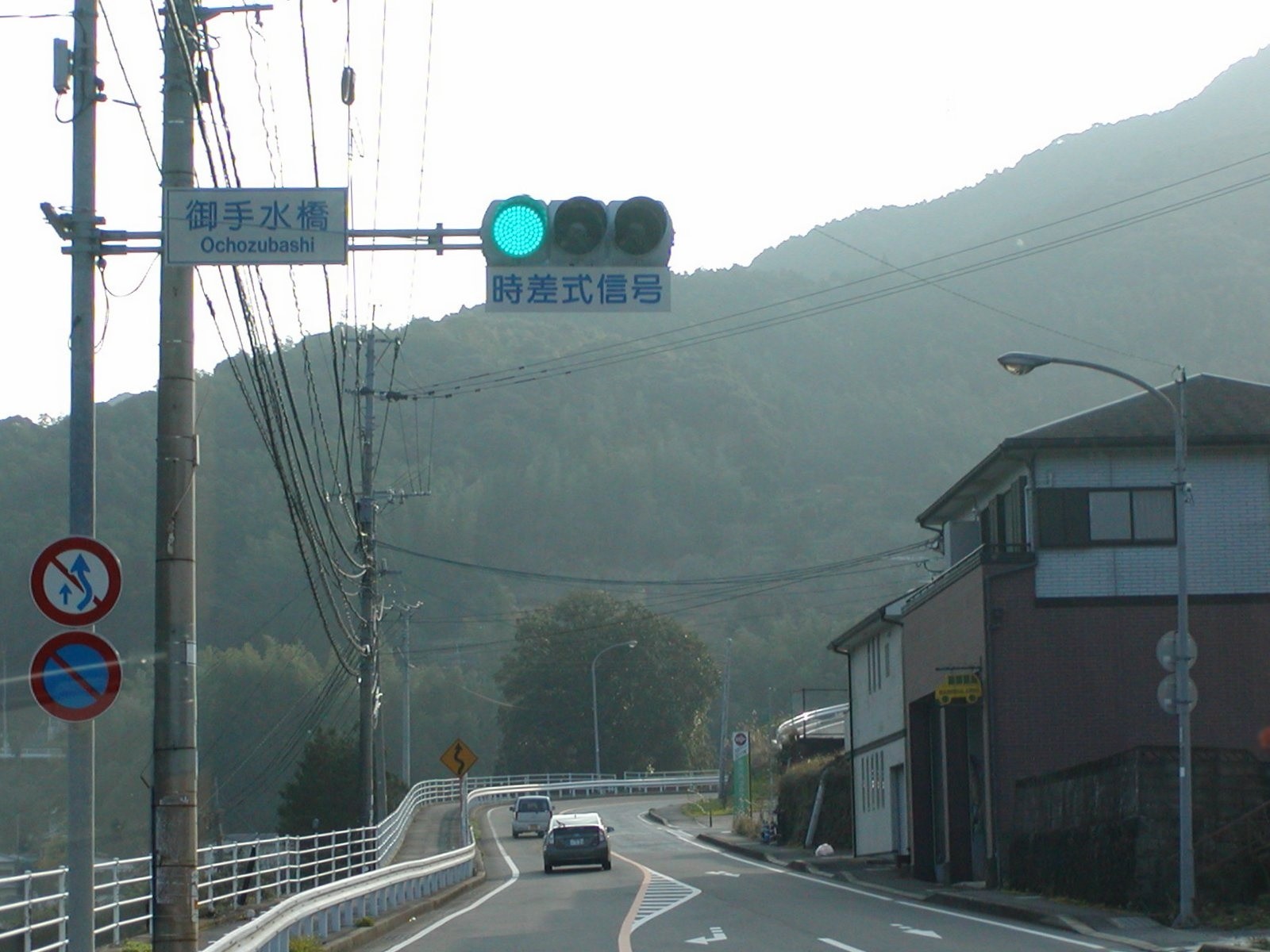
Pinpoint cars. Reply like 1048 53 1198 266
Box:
509 795 556 839
537 811 614 874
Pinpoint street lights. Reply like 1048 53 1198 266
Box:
996 350 1197 930
592 639 639 780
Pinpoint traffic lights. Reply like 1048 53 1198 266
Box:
478 194 675 269
